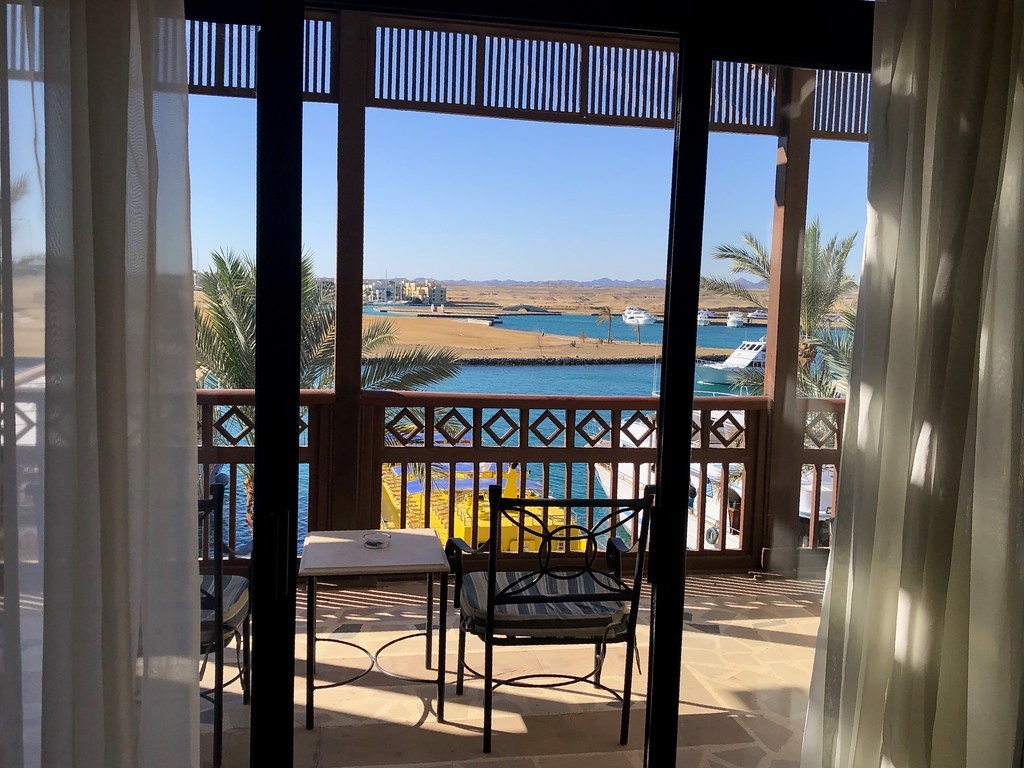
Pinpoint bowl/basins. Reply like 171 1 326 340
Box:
362 532 391 549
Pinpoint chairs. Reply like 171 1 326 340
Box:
193 473 249 768
443 484 654 753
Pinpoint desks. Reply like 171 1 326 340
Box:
296 529 451 729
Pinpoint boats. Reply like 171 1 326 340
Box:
747 310 768 319
707 311 718 318
583 411 838 550
651 354 766 429
697 311 710 326
694 335 766 385
622 307 645 321
625 311 657 324
822 314 841 322
378 425 598 552
726 311 744 327
